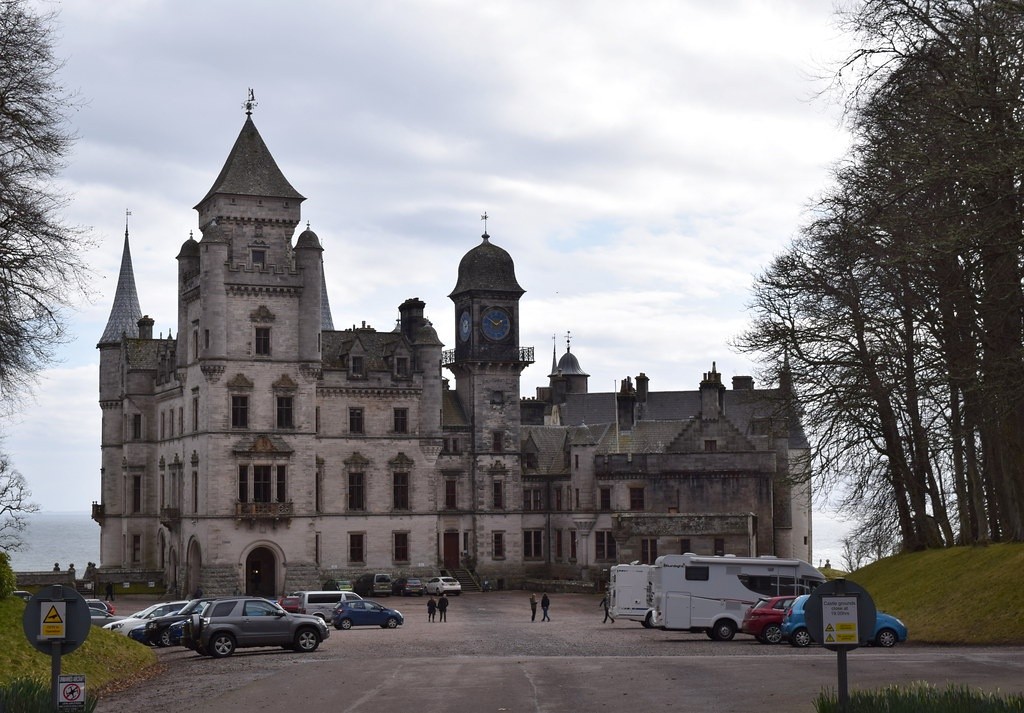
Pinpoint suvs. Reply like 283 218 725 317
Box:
189 598 330 658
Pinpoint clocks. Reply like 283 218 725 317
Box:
458 307 471 343
481 309 511 340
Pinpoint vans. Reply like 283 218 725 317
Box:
353 573 393 597
298 590 375 628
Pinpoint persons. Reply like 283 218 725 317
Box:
529 593 538 621
193 586 202 599
438 593 449 622
599 596 614 624
427 596 437 622
541 594 550 621
105 582 114 601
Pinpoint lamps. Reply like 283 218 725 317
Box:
610 513 622 522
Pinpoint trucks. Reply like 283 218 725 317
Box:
607 561 658 628
647 552 829 642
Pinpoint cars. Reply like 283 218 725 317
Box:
740 596 800 645
10 590 33 603
780 594 908 648
333 600 404 630
391 577 423 596
423 577 462 597
84 596 271 651
279 591 305 614
